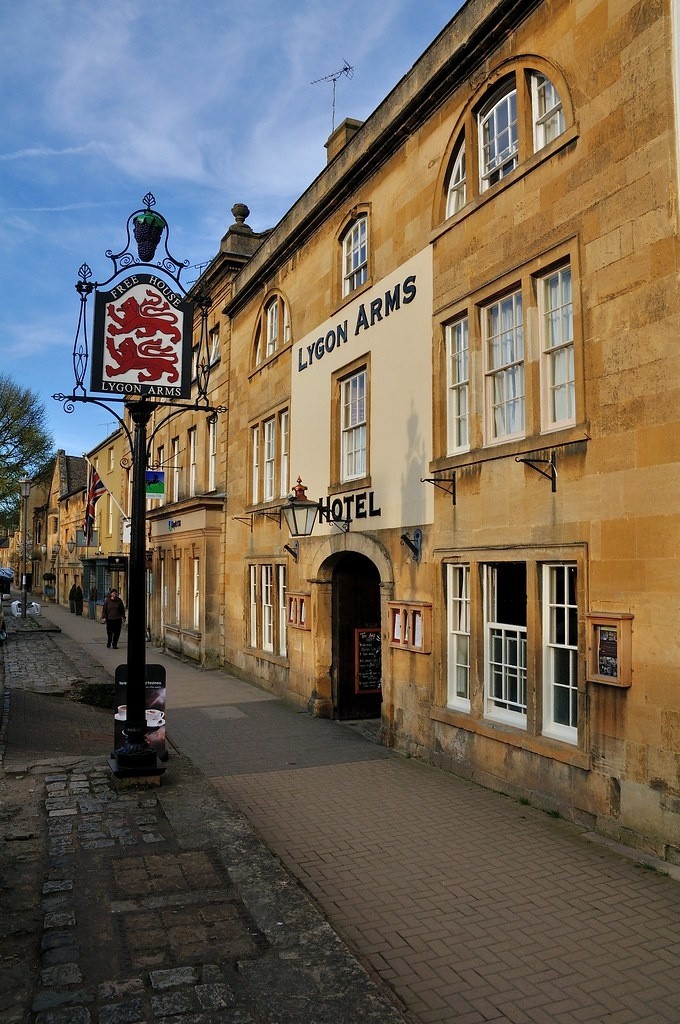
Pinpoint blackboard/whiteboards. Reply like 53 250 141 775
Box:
355 628 382 693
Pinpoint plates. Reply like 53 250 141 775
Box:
146 719 166 727
114 713 126 721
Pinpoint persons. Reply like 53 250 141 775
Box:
102 589 126 649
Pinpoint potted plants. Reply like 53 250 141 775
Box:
88 586 97 620
42 573 56 596
74 586 83 615
68 584 76 613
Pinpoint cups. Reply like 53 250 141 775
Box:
145 709 164 725
118 705 127 719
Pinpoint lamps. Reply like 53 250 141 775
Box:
280 474 354 537
41 543 46 555
54 541 61 554
66 538 76 554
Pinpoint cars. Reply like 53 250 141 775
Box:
0 592 10 641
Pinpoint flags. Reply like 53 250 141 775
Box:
84 460 109 547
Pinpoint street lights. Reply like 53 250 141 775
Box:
18 473 32 618
49 193 233 788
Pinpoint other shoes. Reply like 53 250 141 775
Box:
107 642 111 648
113 645 119 650
